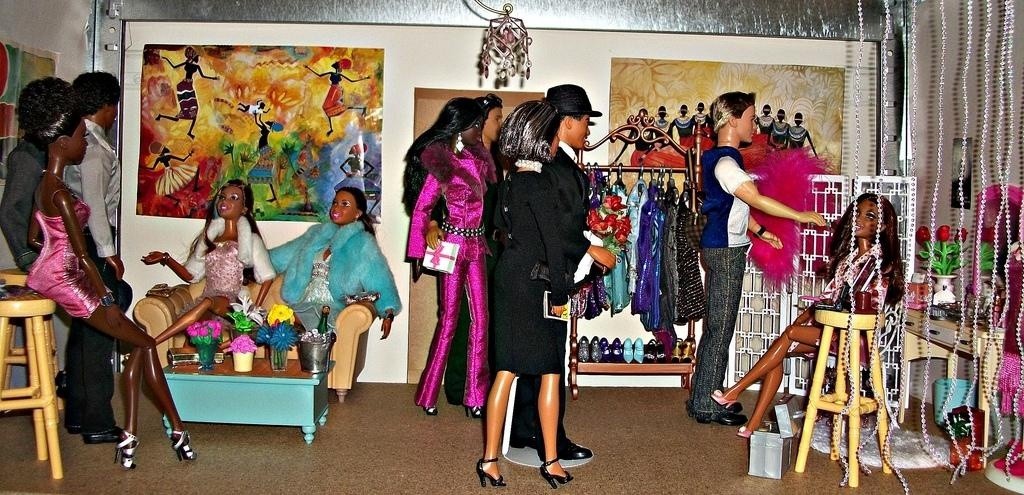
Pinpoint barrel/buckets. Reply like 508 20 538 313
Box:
932 378 976 427
294 338 336 377
